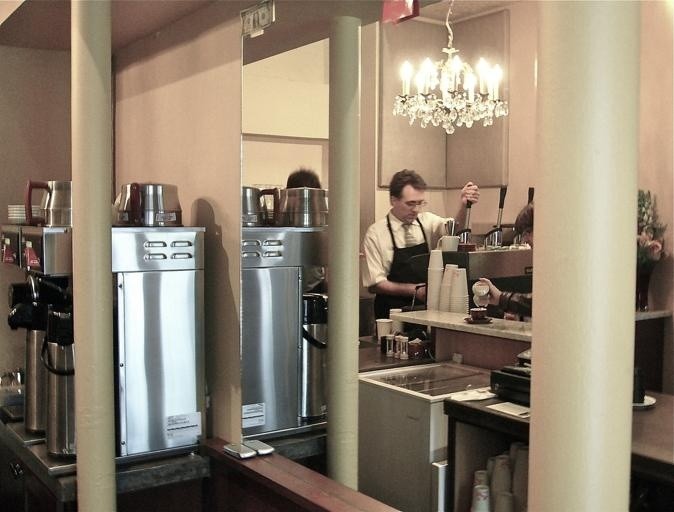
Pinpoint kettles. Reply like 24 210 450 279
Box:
24 179 80 228
242 187 279 226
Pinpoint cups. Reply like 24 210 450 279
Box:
0 366 25 386
427 249 469 315
437 235 460 250
471 307 487 321
470 441 529 512
376 309 403 341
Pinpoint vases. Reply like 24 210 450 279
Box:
634 259 657 312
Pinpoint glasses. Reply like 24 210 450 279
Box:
522 230 533 242
406 200 427 208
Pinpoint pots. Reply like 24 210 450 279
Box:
117 184 185 227
280 188 327 227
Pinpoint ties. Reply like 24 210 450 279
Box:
402 223 416 248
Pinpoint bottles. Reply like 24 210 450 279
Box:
473 282 492 308
386 334 422 359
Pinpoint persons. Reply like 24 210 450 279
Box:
361 169 480 332
478 200 533 319
287 170 329 293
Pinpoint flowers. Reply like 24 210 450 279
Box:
637 187 671 262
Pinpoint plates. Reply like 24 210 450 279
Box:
7 205 41 225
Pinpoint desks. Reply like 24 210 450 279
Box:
444 391 673 511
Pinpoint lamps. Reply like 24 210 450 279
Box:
392 0 509 135
